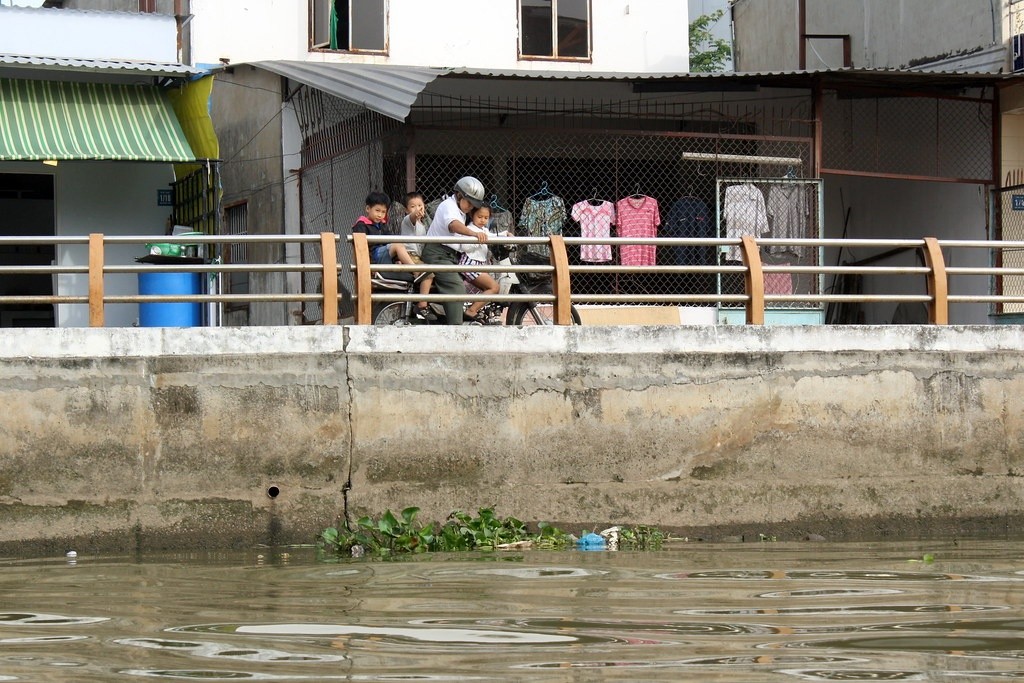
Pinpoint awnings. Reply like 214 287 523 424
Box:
0 76 198 163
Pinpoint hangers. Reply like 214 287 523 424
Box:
617 182 658 204
526 180 563 202
573 186 614 210
772 165 804 183
423 188 453 208
673 184 706 207
488 193 512 214
725 173 762 192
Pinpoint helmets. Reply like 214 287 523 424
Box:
454 176 485 209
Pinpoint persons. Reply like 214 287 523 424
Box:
400 191 438 321
420 175 488 325
351 191 432 282
458 200 515 322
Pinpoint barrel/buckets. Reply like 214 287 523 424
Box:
137 267 201 327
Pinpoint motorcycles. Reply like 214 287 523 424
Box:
371 219 582 326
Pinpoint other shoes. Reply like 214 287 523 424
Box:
412 304 437 320
463 313 489 325
414 271 432 284
